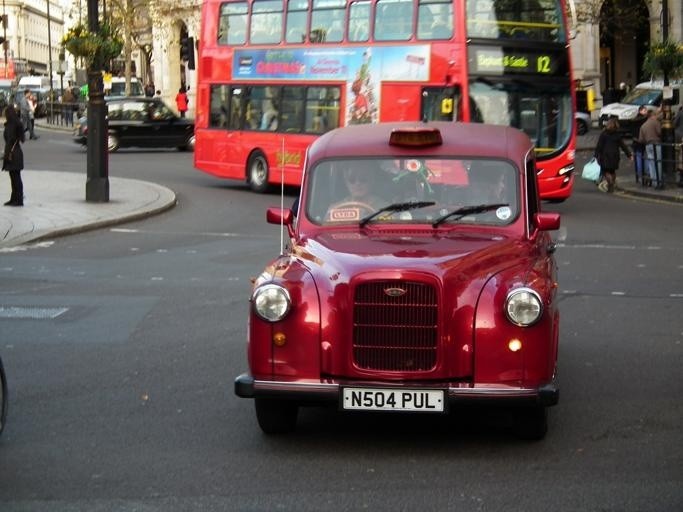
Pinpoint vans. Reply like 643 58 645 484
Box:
0 75 74 116
598 79 682 136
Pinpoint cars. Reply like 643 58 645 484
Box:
521 99 592 137
73 96 194 153
234 120 560 440
103 76 145 99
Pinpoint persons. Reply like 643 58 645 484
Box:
0 82 87 153
2 105 24 207
592 101 682 195
174 87 189 119
463 161 508 222
322 159 400 219
142 81 162 99
207 86 342 135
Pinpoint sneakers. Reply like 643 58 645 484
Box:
4 201 24 206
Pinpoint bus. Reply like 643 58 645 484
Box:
194 1 578 203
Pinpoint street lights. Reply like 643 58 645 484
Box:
55 55 66 99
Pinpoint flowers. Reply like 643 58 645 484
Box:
58 22 124 84
639 41 681 84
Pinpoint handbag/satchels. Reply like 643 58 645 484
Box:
6 151 15 163
581 157 602 181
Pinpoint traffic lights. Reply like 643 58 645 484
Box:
177 38 194 71
